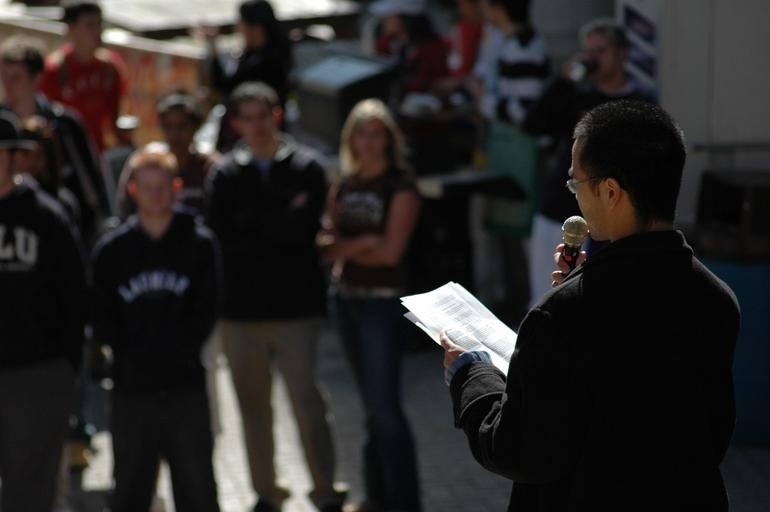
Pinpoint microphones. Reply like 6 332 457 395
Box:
561 215 588 278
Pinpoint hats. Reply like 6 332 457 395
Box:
1 118 33 150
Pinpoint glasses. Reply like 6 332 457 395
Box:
565 174 600 193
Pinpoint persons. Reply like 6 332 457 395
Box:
1 0 426 511
441 100 743 510
378 0 661 322
